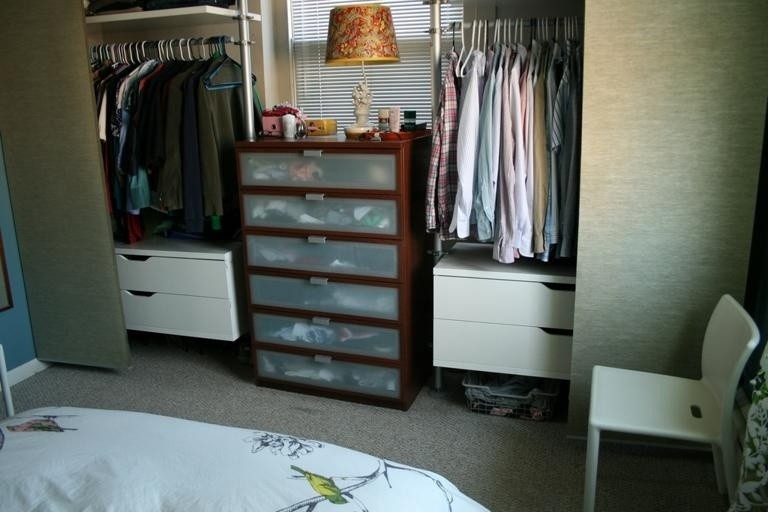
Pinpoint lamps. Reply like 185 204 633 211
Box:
325 3 400 139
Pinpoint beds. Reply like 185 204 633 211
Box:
0 345 493 512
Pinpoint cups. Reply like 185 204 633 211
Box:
282 114 296 139
377 106 401 134
403 110 416 131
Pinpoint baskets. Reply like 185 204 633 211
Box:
462 376 559 422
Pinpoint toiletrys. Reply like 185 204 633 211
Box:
405 111 416 130
379 108 389 132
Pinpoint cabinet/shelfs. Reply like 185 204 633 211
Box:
233 128 432 413
114 237 251 342
431 241 576 392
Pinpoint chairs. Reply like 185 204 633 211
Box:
582 293 761 511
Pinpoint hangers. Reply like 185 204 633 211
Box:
89 34 258 91
447 16 581 80
